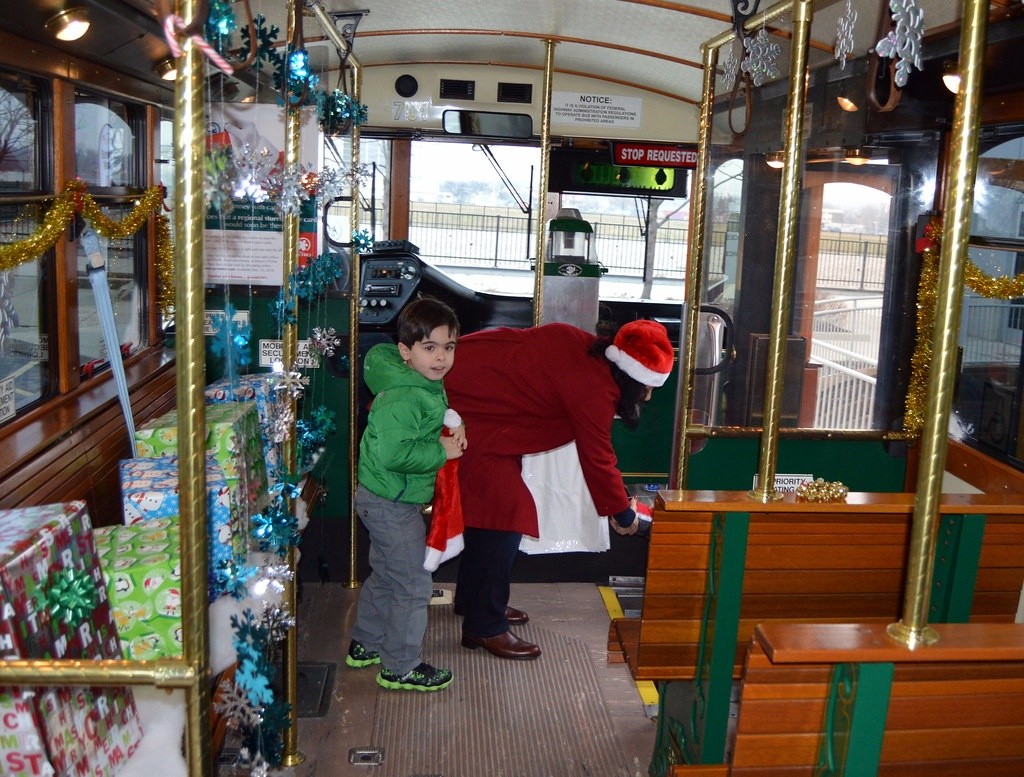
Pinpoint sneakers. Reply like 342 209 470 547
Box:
345 639 381 667
376 662 454 691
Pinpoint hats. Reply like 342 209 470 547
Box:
423 408 465 571
605 320 674 387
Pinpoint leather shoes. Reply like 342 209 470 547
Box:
461 631 541 660
454 603 529 625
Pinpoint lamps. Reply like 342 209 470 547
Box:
43 7 92 42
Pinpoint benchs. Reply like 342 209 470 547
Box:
669 622 1024 777
607 488 1024 777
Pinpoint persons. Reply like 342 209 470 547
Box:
345 300 468 692
444 320 673 660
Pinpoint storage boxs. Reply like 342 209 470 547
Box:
0 498 142 777
92 519 182 661
120 456 234 594
135 401 268 564
204 372 284 479
